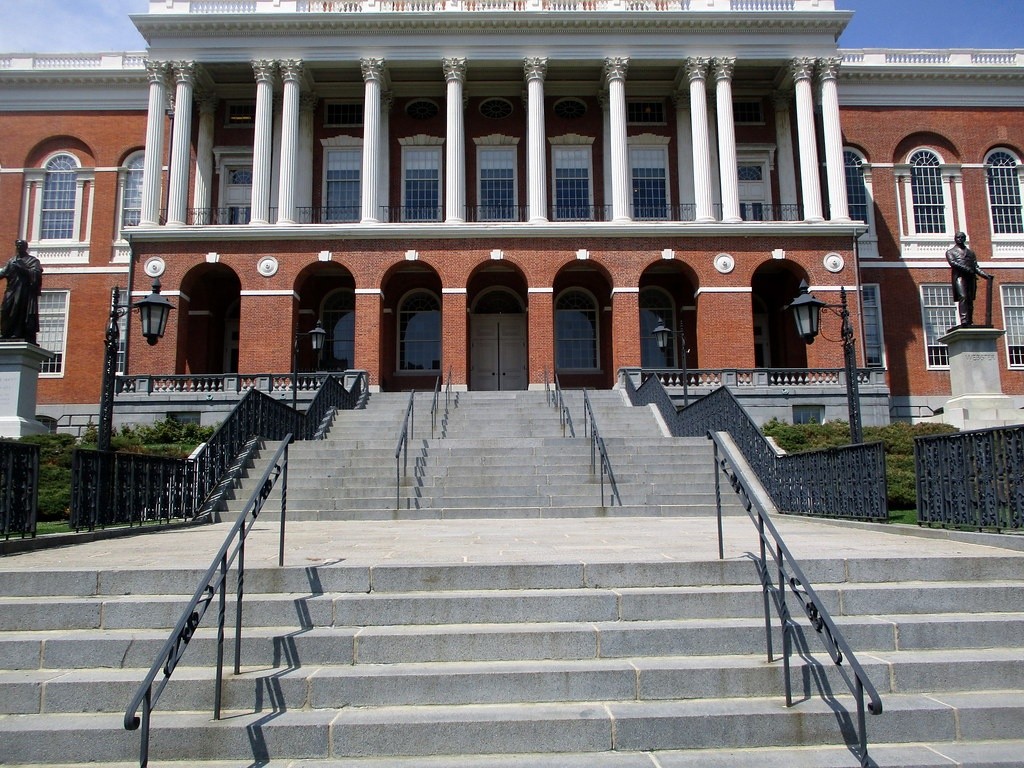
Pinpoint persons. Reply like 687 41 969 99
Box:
0 238 45 343
945 231 993 326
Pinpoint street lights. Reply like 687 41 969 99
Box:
291 319 326 411
784 277 864 447
652 318 689 408
93 279 178 452
